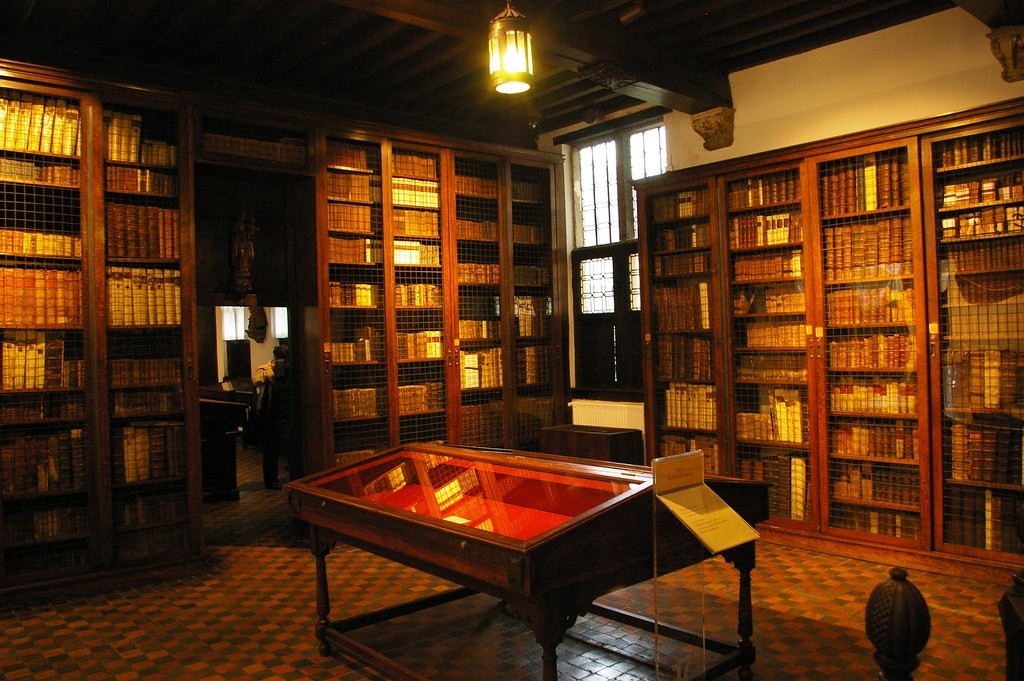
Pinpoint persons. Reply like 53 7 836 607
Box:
251 344 301 484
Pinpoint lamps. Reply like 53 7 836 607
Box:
486 2 535 94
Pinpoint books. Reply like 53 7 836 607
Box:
390 153 445 447
726 169 812 526
938 130 1024 558
455 174 554 447
326 139 384 468
0 89 88 576
102 108 188 562
821 153 922 541
652 186 719 475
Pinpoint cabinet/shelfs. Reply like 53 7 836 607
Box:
618 96 1024 585
0 57 208 612
304 124 566 465
